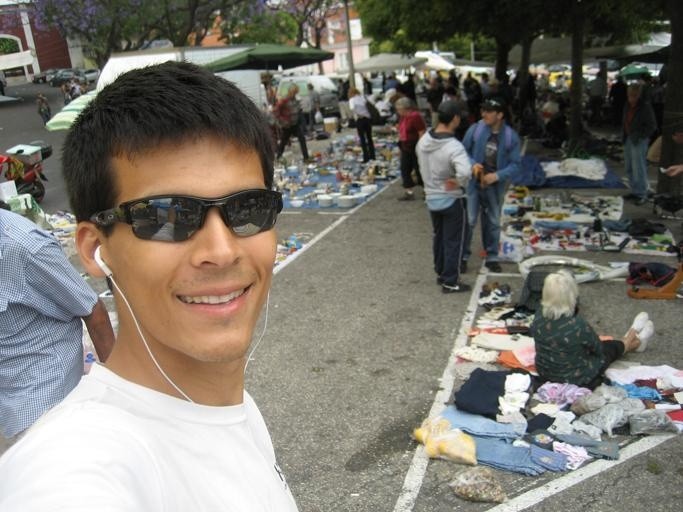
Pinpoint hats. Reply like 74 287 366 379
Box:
438 100 469 117
479 96 506 110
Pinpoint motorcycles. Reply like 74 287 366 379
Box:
33 90 50 123
0 139 52 203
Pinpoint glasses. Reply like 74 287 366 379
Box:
92 188 283 244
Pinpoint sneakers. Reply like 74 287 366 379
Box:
484 260 503 274
442 280 472 294
631 311 648 331
634 320 654 353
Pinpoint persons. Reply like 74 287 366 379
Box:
256 70 682 207
532 270 653 389
414 100 472 292
0 61 303 511
459 98 521 272
0 207 115 455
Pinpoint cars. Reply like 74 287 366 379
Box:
32 67 100 84
527 61 628 91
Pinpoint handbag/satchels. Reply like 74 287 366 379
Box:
366 101 386 126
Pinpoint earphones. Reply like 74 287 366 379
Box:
94 245 114 278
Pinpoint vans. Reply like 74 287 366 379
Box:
275 76 338 116
